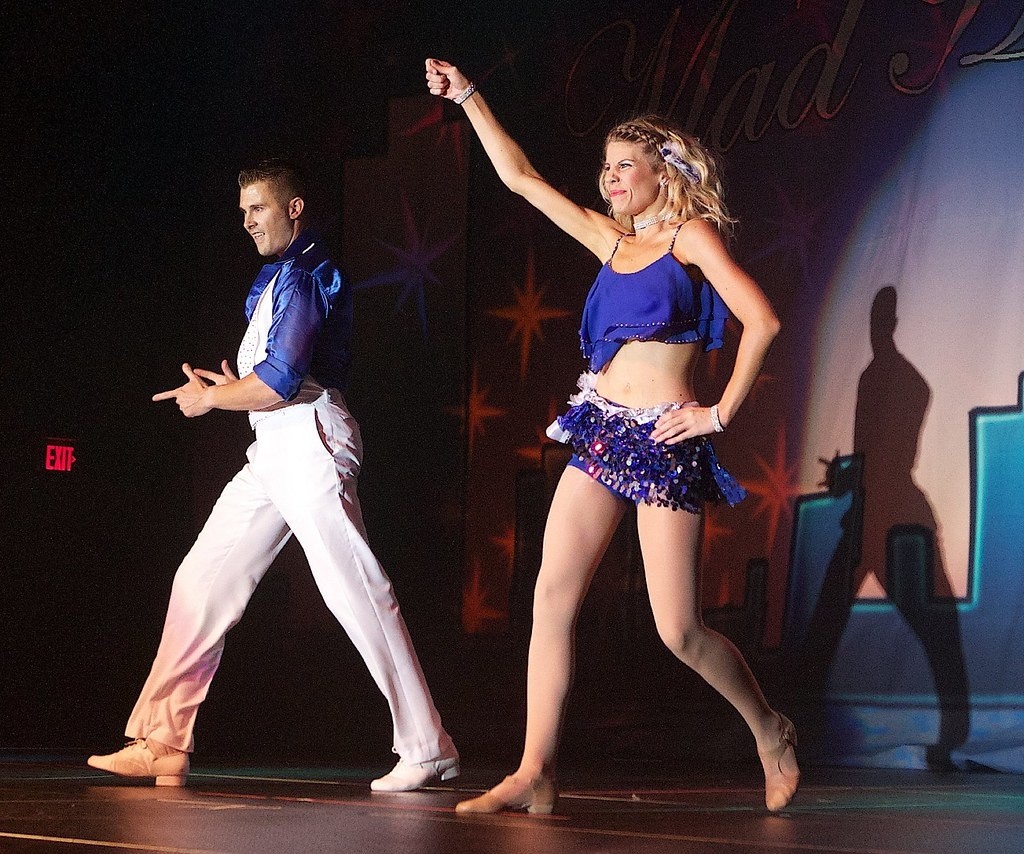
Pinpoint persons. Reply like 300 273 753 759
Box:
425 56 804 813
88 158 461 792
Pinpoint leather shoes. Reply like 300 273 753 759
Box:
372 743 461 790
87 739 189 786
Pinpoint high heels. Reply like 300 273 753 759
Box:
756 713 800 811
457 776 557 815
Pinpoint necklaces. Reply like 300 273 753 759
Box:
633 208 677 230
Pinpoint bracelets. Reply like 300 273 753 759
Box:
453 81 476 105
711 404 724 432
